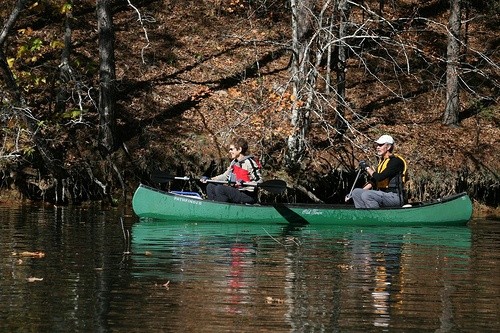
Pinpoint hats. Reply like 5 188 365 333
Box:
374 135 394 145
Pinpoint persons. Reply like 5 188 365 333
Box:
200 136 263 205
344 135 407 209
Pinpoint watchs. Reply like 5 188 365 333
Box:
366 167 370 170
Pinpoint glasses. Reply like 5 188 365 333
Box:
378 144 384 146
228 148 236 151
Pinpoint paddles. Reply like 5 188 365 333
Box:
344 168 363 205
169 177 287 193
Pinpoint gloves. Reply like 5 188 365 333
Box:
359 160 370 171
344 194 353 202
200 176 210 183
239 179 247 187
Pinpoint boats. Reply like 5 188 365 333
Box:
132 183 473 227
